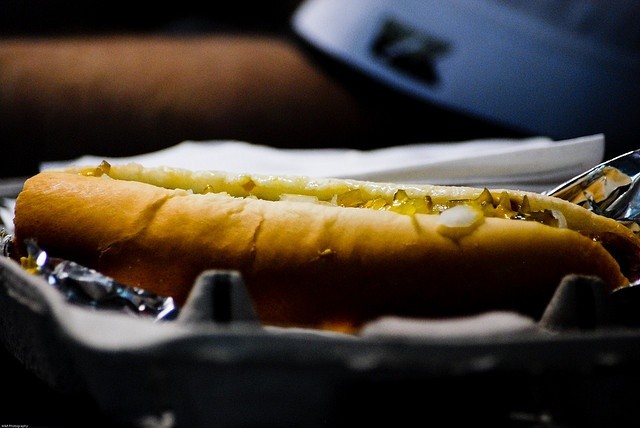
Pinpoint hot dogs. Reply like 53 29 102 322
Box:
13 160 635 331
